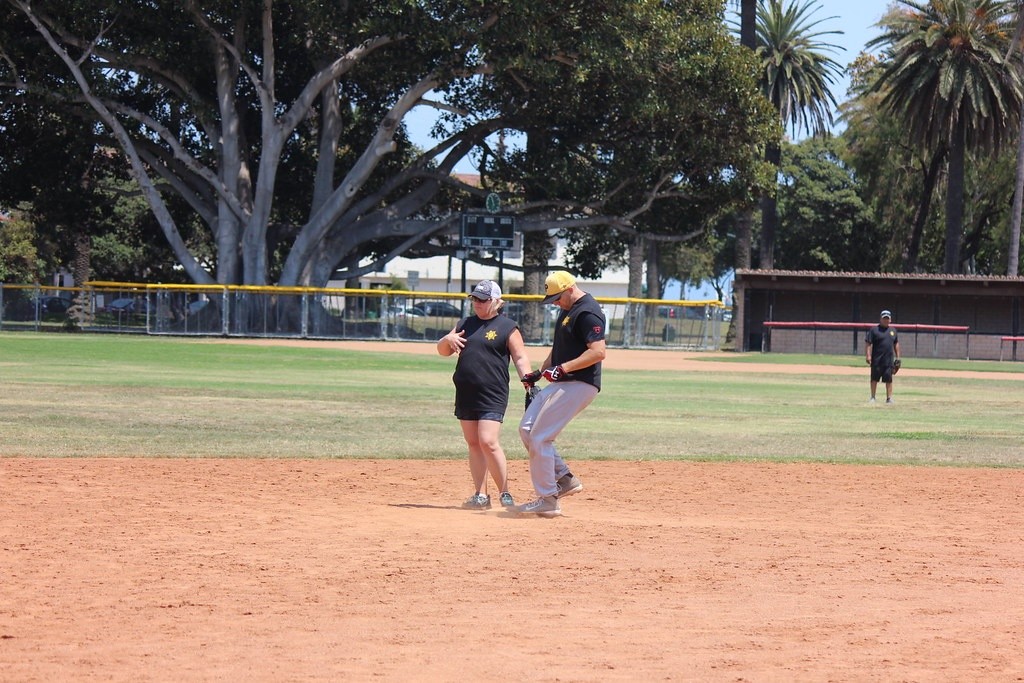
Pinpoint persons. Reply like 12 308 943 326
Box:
519 270 606 513
866 311 901 403
438 280 541 510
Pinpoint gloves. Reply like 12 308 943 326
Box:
540 365 568 382
521 368 544 382
523 382 541 399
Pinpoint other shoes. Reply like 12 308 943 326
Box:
870 397 875 402
886 398 893 403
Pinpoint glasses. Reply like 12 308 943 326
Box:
552 295 561 304
468 297 492 303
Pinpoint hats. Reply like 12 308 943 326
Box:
880 310 891 318
542 270 577 304
468 280 501 300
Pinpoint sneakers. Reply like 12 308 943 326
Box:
556 472 583 497
519 495 561 513
463 491 492 509
500 492 514 507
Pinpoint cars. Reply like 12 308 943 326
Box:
388 305 429 319
414 300 461 317
181 300 210 327
17 295 74 321
660 304 733 321
539 305 561 324
95 298 156 323
501 303 523 328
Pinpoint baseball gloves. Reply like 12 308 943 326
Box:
892 359 902 375
524 386 542 412
